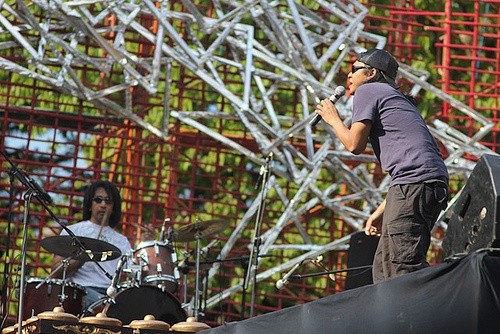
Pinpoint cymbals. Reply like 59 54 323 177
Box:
165 219 230 242
41 235 122 262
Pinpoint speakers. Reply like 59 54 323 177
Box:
440 152 500 258
344 228 380 292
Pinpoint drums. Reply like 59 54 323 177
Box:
23 277 85 321
133 241 178 293
86 284 187 329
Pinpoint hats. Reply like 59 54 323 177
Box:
358 49 400 89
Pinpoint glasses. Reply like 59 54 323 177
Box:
91 196 114 204
352 65 371 74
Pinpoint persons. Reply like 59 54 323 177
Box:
51 179 134 310
316 48 449 284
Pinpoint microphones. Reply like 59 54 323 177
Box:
201 238 218 254
309 85 346 127
106 258 123 297
275 259 304 291
29 178 56 207
159 217 168 241
